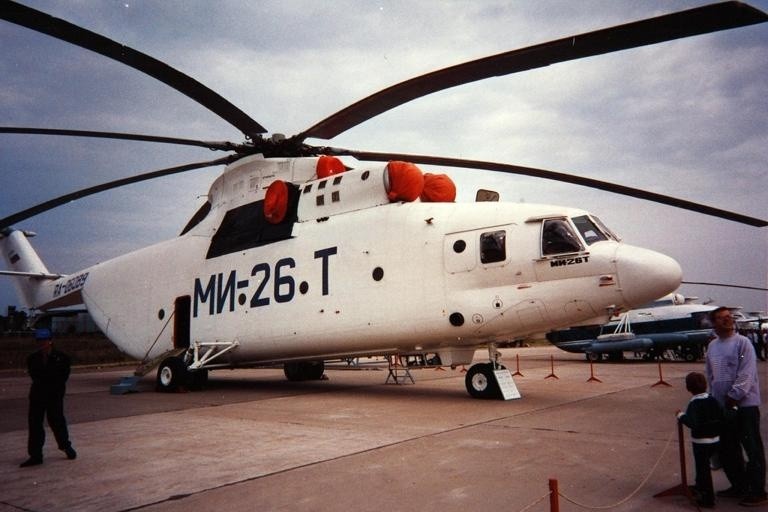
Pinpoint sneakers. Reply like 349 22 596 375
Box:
691 497 715 508
65 445 76 460
689 485 714 495
740 491 768 506
717 487 749 498
20 456 43 467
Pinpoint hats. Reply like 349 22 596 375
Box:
35 328 50 339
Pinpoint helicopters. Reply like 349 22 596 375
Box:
544 291 768 368
0 1 768 402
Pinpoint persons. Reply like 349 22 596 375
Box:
18 328 78 468
671 372 749 510
700 306 768 507
707 327 768 362
